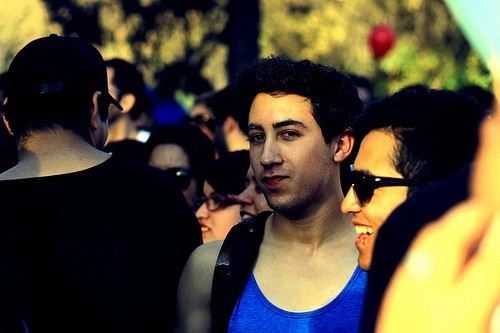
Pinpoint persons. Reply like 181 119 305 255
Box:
0 33 204 333
102 57 272 242
340 84 495 333
178 53 368 333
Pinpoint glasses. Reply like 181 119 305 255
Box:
195 190 241 211
184 115 215 129
343 170 410 201
168 167 195 189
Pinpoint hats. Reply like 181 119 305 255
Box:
6 34 124 110
